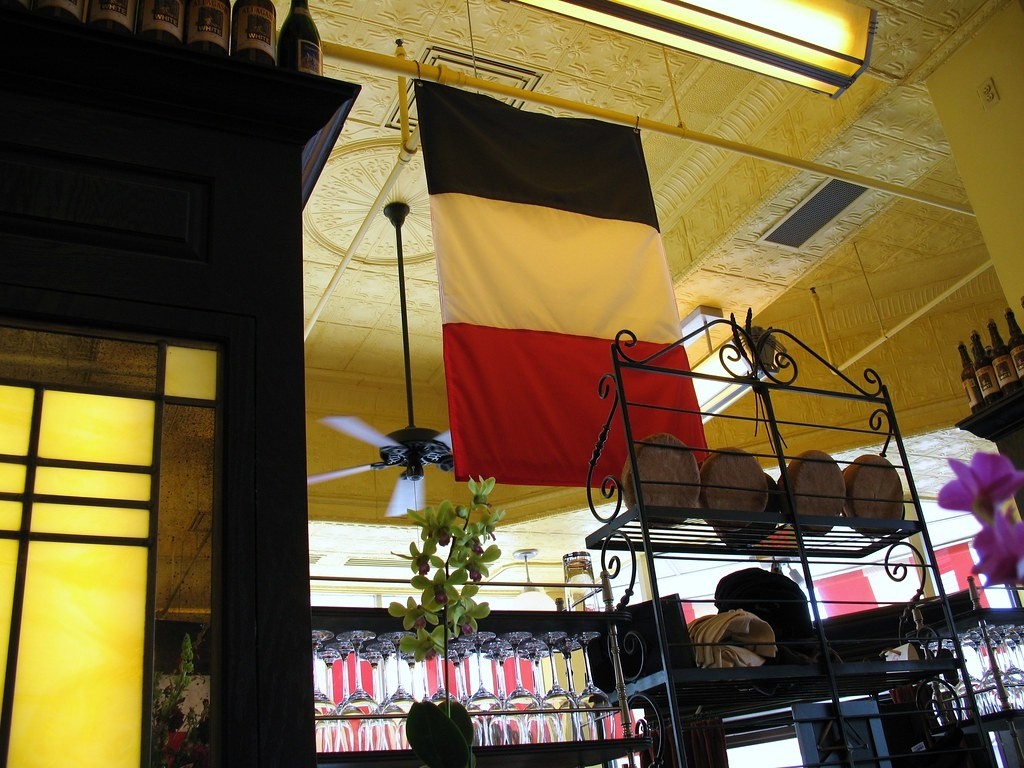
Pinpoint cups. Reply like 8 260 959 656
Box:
563 552 596 612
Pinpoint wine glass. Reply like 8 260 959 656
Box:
920 623 1024 727
313 629 616 753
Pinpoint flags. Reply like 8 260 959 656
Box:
413 77 710 490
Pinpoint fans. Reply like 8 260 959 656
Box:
308 201 453 518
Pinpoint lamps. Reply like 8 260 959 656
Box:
506 0 878 101
514 550 558 610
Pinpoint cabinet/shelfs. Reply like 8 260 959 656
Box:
586 309 1024 768
311 570 670 767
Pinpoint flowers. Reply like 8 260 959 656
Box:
935 452 1024 590
386 475 502 768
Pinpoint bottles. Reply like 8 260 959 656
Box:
957 340 986 413
136 0 183 45
970 330 993 406
231 0 275 68
277 0 323 77
88 0 138 34
984 317 1017 396
183 0 231 58
1020 296 1024 307
1003 307 1024 382
0 0 86 21
